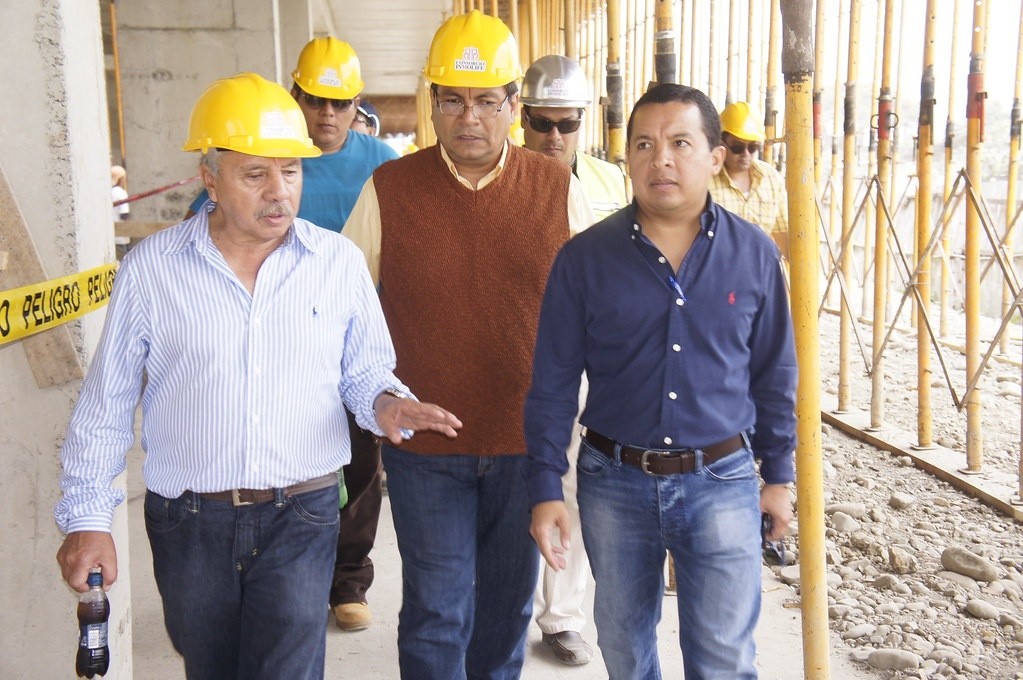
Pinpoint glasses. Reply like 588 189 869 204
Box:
437 95 508 119
760 511 786 567
720 138 759 154
353 115 370 127
300 93 360 112
524 111 581 134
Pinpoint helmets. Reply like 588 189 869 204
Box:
181 72 323 159
424 9 522 88
292 36 364 100
720 101 767 142
519 55 592 108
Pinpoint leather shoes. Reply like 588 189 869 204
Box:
542 631 593 665
331 601 370 630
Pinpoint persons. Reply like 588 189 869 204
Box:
338 13 596 680
57 72 461 680
183 37 401 630
518 56 632 665
110 164 132 261
708 101 789 260
524 84 798 680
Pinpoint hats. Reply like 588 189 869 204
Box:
355 100 381 138
110 165 126 186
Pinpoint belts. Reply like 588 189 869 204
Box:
183 473 337 507
586 427 751 478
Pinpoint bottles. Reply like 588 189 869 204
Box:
74 573 110 679
